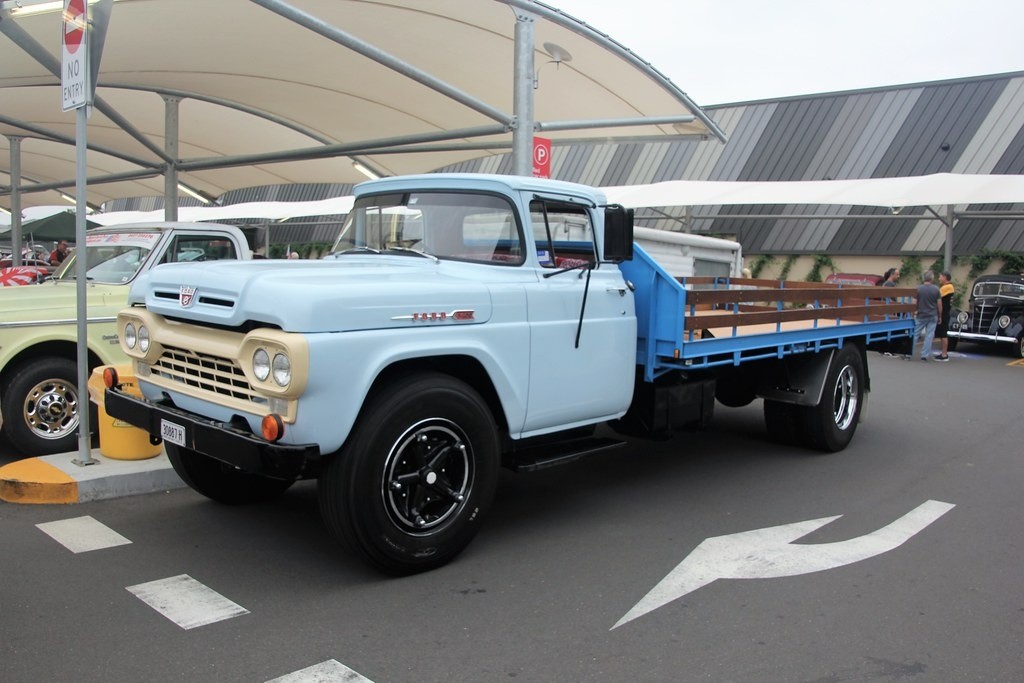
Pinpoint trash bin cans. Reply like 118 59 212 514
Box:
90 363 163 459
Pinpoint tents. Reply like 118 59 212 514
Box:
0 211 102 284
86 175 1024 259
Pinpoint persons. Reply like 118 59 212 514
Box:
882 268 900 357
32 251 41 259
901 270 942 363
50 240 67 266
934 271 954 361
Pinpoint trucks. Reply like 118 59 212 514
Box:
0 221 255 457
323 204 744 290
101 172 918 580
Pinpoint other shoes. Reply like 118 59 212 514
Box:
935 353 948 360
904 354 911 361
884 352 898 358
922 357 926 361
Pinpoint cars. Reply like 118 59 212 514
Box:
824 271 911 331
947 274 1024 360
0 246 60 284
